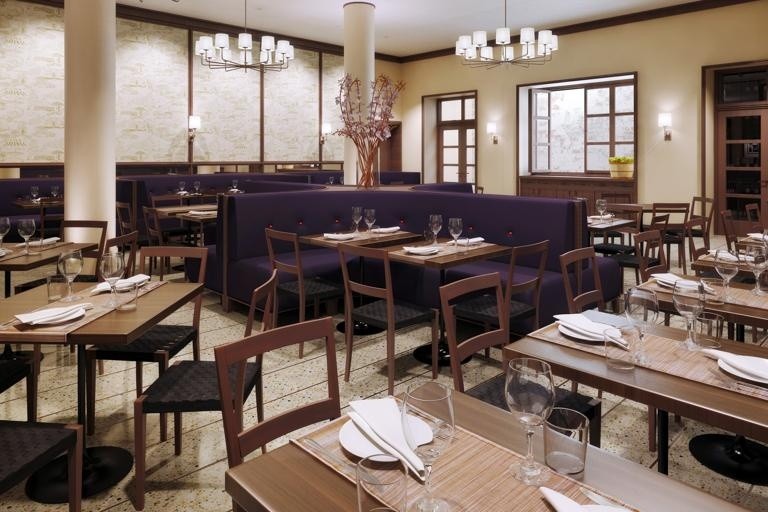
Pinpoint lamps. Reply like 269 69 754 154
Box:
455 1 558 69
486 122 499 145
195 1 294 74
188 114 200 142
659 113 672 141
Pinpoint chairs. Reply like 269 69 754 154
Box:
264 225 340 360
686 217 709 276
215 315 341 470
594 202 642 255
668 197 717 263
134 268 279 512
103 230 138 280
632 229 669 322
649 201 690 275
745 203 763 233
439 270 602 450
721 209 740 253
559 246 607 399
0 417 84 512
0 349 44 423
13 218 108 295
454 240 552 366
116 202 157 276
609 213 671 296
337 242 440 397
86 244 210 436
142 205 190 272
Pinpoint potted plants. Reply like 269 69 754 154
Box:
608 156 634 178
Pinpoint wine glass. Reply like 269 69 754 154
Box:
745 236 768 296
596 199 608 224
429 214 442 246
17 219 35 252
194 181 201 195
505 358 554 486
57 249 84 301
401 381 463 512
30 186 39 201
232 179 239 194
625 287 659 347
351 207 363 234
364 209 376 234
179 181 186 197
101 252 123 305
448 218 462 249
50 185 58 197
673 279 704 351
330 177 334 187
0 218 10 256
714 250 738 298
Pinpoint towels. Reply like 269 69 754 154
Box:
707 249 737 260
187 210 216 216
590 214 611 218
367 226 400 234
746 233 768 237
16 236 61 248
703 348 768 380
650 273 716 295
323 232 362 240
540 487 582 511
447 236 485 243
347 395 427 482
403 245 444 251
14 303 95 326
552 314 630 351
89 273 150 294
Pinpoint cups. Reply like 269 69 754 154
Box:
603 329 637 371
113 280 137 312
358 454 407 512
544 407 589 478
696 313 725 348
47 274 68 304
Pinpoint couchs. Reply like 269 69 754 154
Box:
0 177 64 242
412 181 622 312
227 190 576 339
116 173 329 306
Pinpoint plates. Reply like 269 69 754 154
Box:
410 249 438 255
454 241 482 246
559 321 622 341
338 412 432 462
657 278 698 291
718 355 768 385
325 234 354 240
34 306 84 325
97 279 135 292
